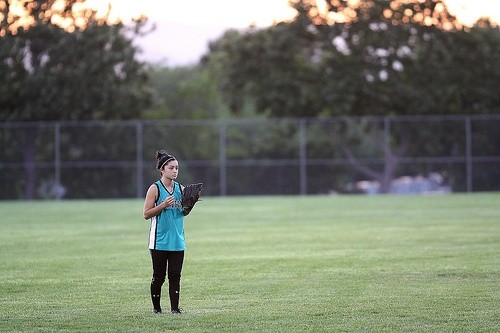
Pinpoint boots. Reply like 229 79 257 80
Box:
151 284 162 313
169 284 181 314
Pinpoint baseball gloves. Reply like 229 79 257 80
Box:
181 183 203 208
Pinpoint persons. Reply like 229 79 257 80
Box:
143 150 202 313
